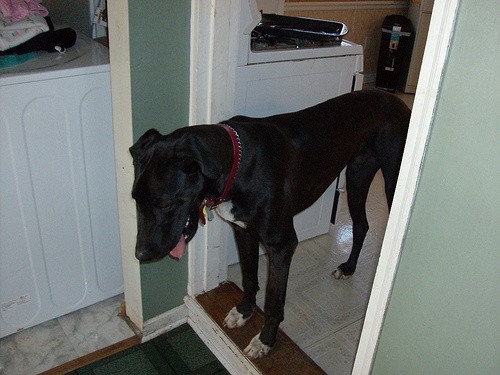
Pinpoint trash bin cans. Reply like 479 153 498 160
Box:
374 14 416 92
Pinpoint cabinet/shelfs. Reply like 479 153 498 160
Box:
228 40 365 266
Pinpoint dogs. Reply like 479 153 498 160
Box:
127 86 413 358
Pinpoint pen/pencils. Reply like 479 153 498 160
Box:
257 44 297 49
